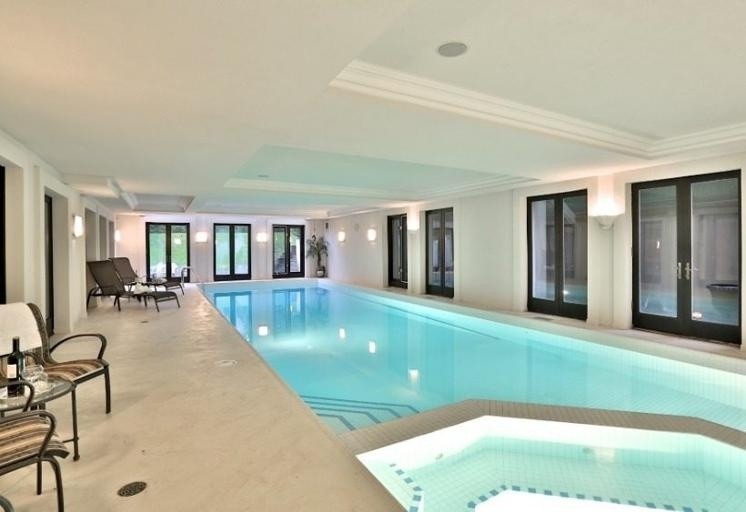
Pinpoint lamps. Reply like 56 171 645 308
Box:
338 327 346 339
258 325 269 336
407 368 420 385
114 230 121 242
337 231 346 243
257 232 269 243
194 232 209 243
366 228 377 242
72 216 85 238
369 340 376 354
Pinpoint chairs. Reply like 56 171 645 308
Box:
85 256 189 315
0 380 82 512
0 301 111 440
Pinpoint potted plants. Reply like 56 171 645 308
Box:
314 288 328 323
306 234 330 277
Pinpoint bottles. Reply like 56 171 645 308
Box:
6 337 25 397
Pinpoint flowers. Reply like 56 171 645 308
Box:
407 210 420 233
593 196 625 229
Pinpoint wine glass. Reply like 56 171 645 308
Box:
22 364 43 383
